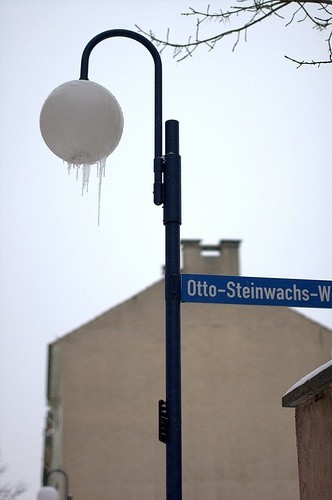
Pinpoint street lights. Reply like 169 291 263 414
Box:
39 28 185 495
